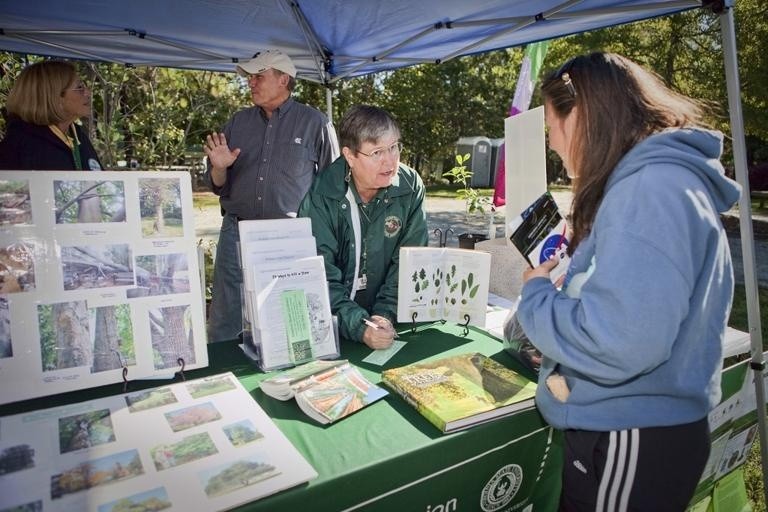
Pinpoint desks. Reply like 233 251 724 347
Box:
0 292 757 512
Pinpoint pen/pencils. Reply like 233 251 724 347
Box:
360 318 400 338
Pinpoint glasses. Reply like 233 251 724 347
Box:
356 142 404 161
66 79 89 91
552 55 577 99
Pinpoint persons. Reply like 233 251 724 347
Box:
516 52 743 511
202 49 341 342
297 105 429 349
0 60 103 170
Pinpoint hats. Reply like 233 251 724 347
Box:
237 49 297 79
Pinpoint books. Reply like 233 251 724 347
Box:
395 245 491 326
258 358 386 427
238 217 335 366
383 353 539 436
